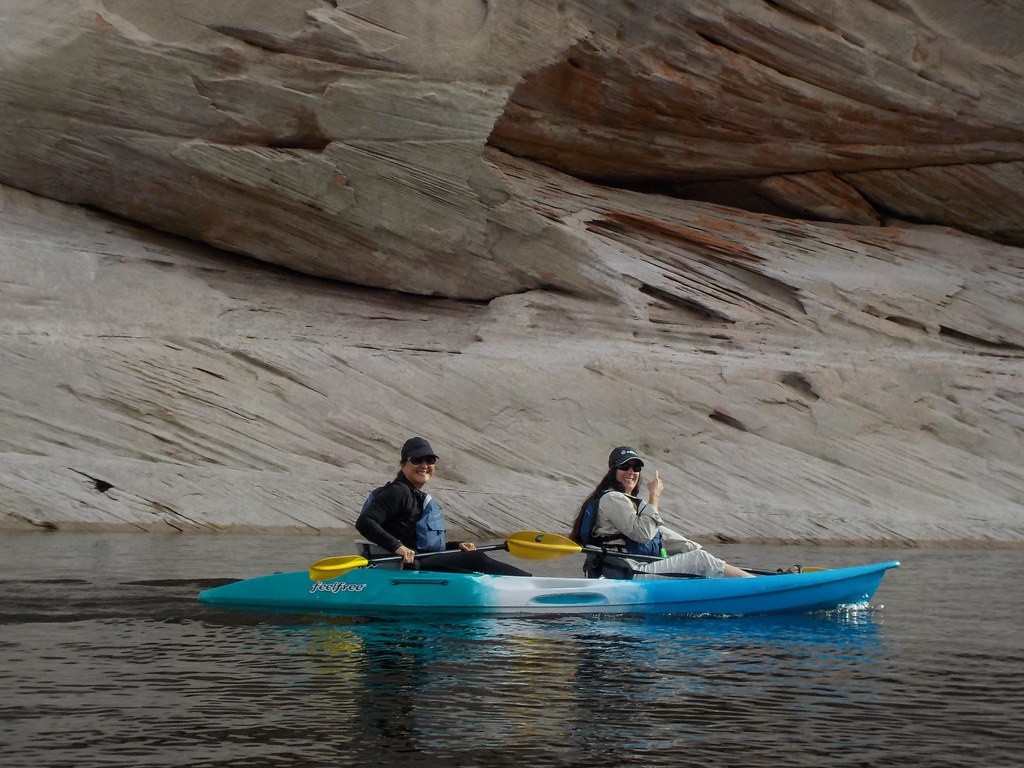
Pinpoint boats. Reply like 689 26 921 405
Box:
197 557 904 616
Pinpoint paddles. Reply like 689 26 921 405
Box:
308 545 503 586
507 531 832 575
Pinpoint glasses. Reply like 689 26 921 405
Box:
405 457 436 465
616 463 641 472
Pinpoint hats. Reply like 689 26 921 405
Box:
402 437 439 458
609 446 644 467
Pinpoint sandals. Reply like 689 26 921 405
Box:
785 565 802 574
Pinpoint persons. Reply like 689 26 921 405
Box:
356 436 531 577
571 444 755 582
